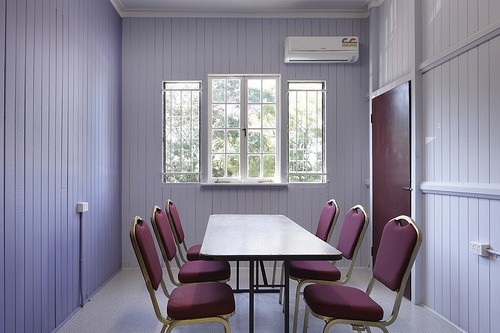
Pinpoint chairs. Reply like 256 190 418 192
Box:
293 214 423 333
271 198 340 289
164 198 202 261
129 215 236 333
278 204 370 304
149 205 231 287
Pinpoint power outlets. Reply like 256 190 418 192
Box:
76 201 89 213
470 240 490 260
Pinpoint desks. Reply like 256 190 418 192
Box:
198 214 343 333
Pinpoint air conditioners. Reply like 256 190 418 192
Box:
283 36 360 64
